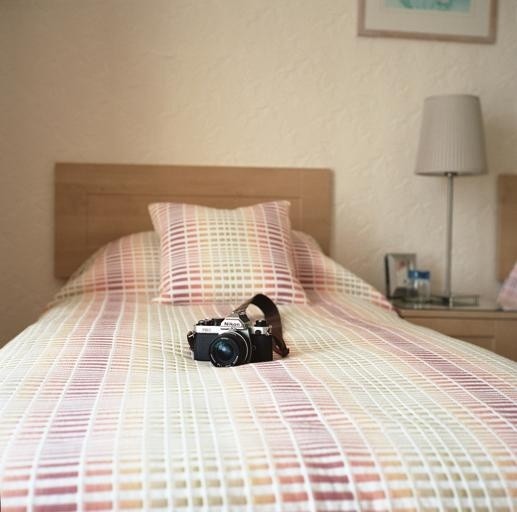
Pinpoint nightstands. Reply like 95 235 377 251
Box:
391 305 515 359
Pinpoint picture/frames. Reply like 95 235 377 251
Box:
383 251 418 299
357 0 499 47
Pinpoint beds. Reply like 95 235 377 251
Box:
0 164 516 512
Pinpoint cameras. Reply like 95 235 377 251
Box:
192 319 273 368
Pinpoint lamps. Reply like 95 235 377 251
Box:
418 93 491 308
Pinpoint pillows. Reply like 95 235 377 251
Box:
148 199 307 307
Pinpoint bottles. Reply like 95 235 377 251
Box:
403 270 432 311
